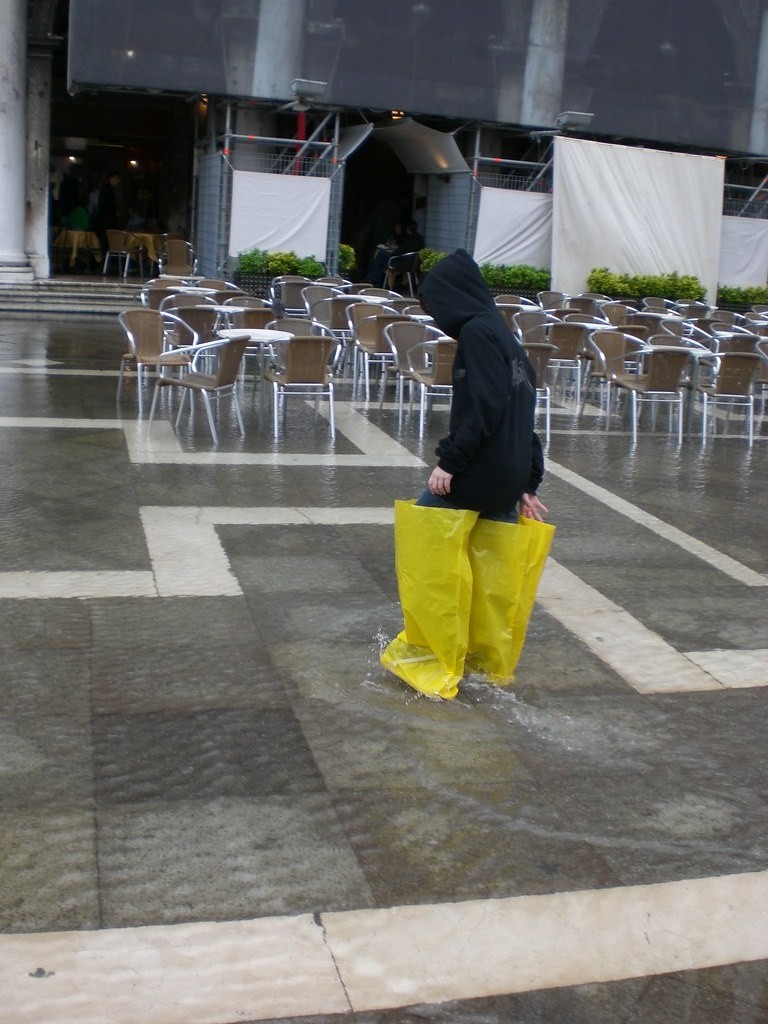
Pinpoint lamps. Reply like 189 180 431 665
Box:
269 78 328 116
529 111 594 138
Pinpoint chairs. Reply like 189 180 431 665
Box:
103 229 144 279
114 233 768 446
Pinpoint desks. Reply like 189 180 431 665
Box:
54 230 101 270
125 233 162 276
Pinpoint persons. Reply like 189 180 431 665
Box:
378 221 425 286
57 164 145 274
380 247 554 700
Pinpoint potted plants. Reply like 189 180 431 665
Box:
232 248 329 300
479 263 551 304
338 242 355 281
716 283 768 313
585 267 707 305
